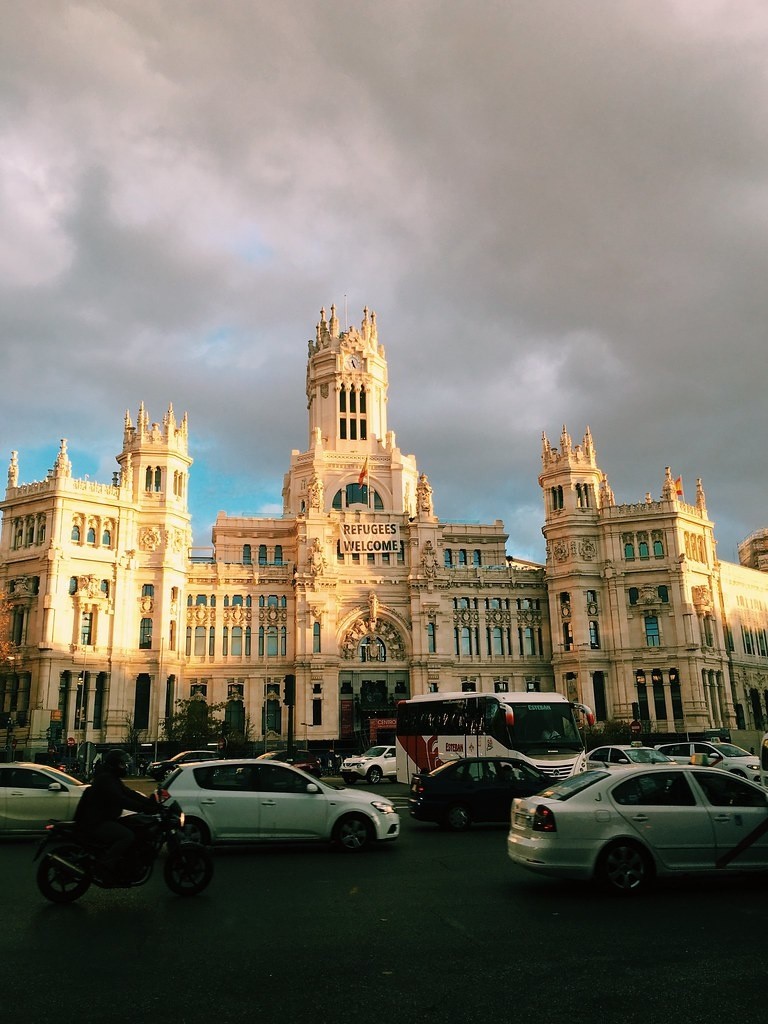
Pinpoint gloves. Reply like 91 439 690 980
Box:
144 797 157 805
144 804 164 814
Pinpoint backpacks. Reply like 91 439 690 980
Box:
73 786 97 822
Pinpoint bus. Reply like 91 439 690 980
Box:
393 690 595 783
653 736 762 785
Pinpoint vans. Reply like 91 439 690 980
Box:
758 733 768 798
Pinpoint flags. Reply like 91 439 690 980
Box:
359 456 368 489
675 475 683 495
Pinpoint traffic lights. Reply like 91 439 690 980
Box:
45 727 53 741
631 701 641 719
55 727 63 740
282 673 297 705
222 720 231 734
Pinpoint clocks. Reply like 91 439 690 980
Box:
344 353 361 371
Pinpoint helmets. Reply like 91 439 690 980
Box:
105 749 132 777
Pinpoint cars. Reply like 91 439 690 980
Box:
584 740 680 788
0 761 147 835
406 755 562 833
505 762 768 901
154 756 401 855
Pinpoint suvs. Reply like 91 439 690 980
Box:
235 748 324 789
146 749 227 781
338 745 398 785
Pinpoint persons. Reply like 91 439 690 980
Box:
750 747 756 754
368 594 380 623
326 748 338 777
54 758 149 779
74 749 164 886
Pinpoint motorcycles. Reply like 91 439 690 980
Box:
31 782 216 906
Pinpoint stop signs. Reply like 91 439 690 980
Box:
630 721 641 734
11 741 17 749
48 747 55 755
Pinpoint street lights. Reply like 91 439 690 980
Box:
300 722 314 750
